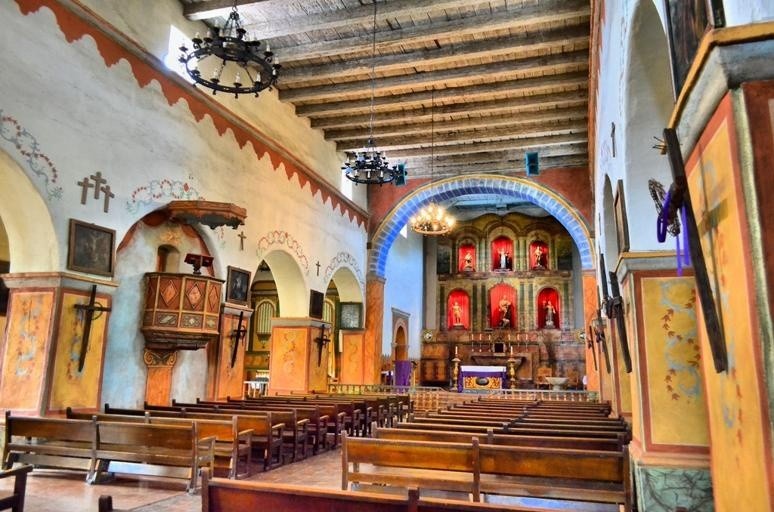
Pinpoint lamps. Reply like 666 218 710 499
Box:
341 0 403 187
409 87 456 237
178 0 282 99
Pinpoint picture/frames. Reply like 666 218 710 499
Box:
613 179 630 258
67 218 116 277
226 265 251 305
309 289 324 319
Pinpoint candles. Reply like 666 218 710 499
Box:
455 333 528 354
